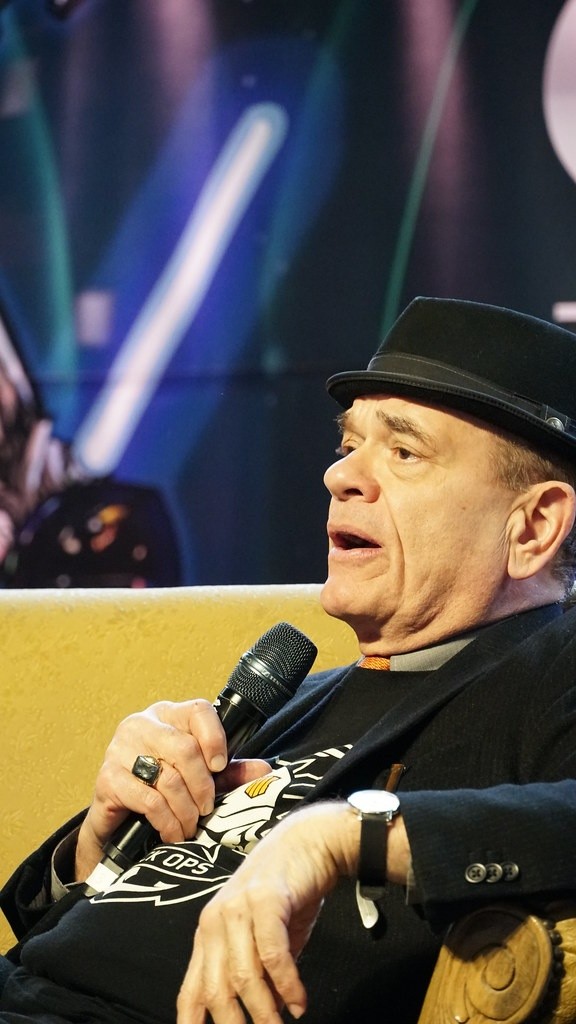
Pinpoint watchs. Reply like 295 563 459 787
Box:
347 789 401 903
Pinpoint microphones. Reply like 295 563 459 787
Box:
85 622 317 895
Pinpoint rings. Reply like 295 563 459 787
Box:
132 754 165 788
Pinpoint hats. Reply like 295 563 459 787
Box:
327 298 576 450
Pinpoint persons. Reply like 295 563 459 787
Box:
0 295 576 1024
